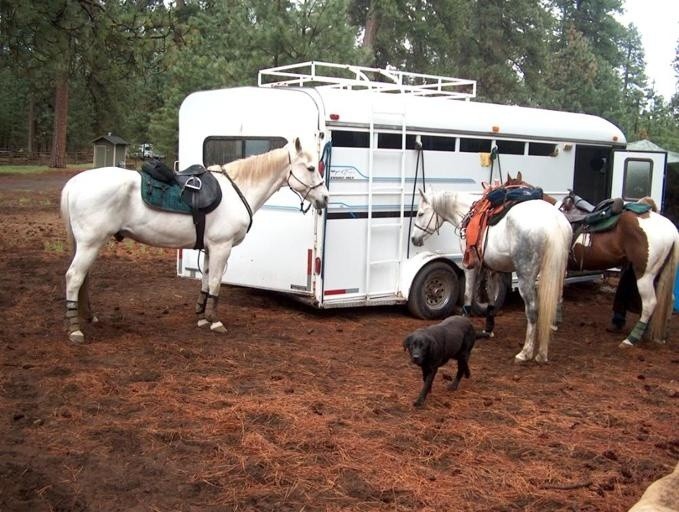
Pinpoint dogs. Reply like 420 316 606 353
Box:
402 315 490 406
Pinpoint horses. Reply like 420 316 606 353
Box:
60 137 330 344
411 183 574 365
503 171 679 350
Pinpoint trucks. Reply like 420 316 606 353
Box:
175 60 668 320
